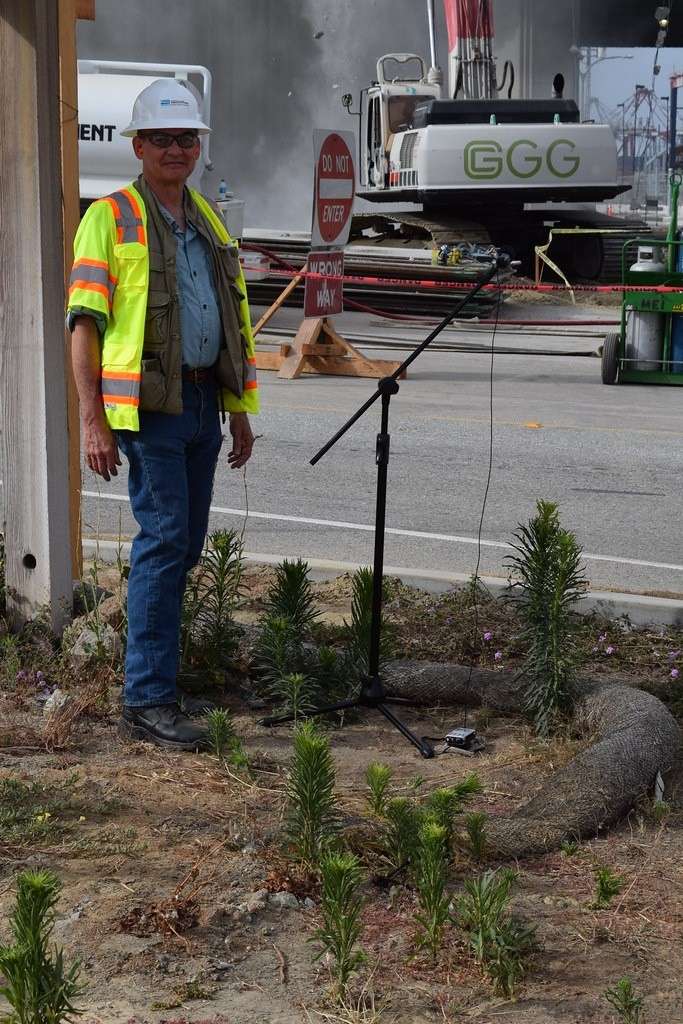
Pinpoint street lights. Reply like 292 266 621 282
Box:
579 54 634 125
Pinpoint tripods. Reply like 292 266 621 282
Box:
257 265 499 759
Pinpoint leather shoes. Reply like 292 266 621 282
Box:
115 704 211 750
174 689 213 715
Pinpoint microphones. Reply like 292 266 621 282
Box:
479 254 511 287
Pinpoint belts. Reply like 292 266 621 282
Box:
186 366 213 384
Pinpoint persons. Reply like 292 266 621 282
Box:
67 79 259 751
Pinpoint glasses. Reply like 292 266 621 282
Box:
141 131 199 148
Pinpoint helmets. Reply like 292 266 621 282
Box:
120 79 213 137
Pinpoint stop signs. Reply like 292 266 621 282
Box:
310 127 355 244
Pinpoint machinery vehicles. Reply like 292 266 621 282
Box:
348 0 660 284
